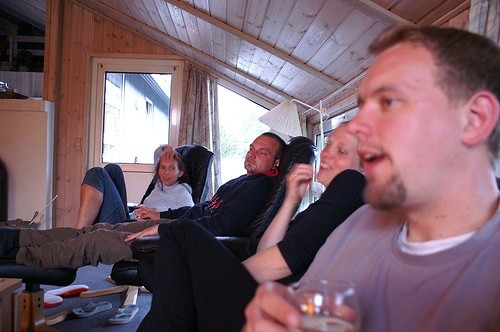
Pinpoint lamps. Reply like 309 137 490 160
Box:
258 99 330 193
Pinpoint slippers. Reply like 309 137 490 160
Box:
109 304 139 323
71 301 111 318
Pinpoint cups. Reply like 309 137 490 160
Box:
287 278 361 332
133 211 144 220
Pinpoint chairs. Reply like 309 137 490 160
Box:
79 143 214 304
111 137 316 287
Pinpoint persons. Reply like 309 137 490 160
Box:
75 146 196 230
240 25 500 331
0 133 287 268
136 121 367 332
